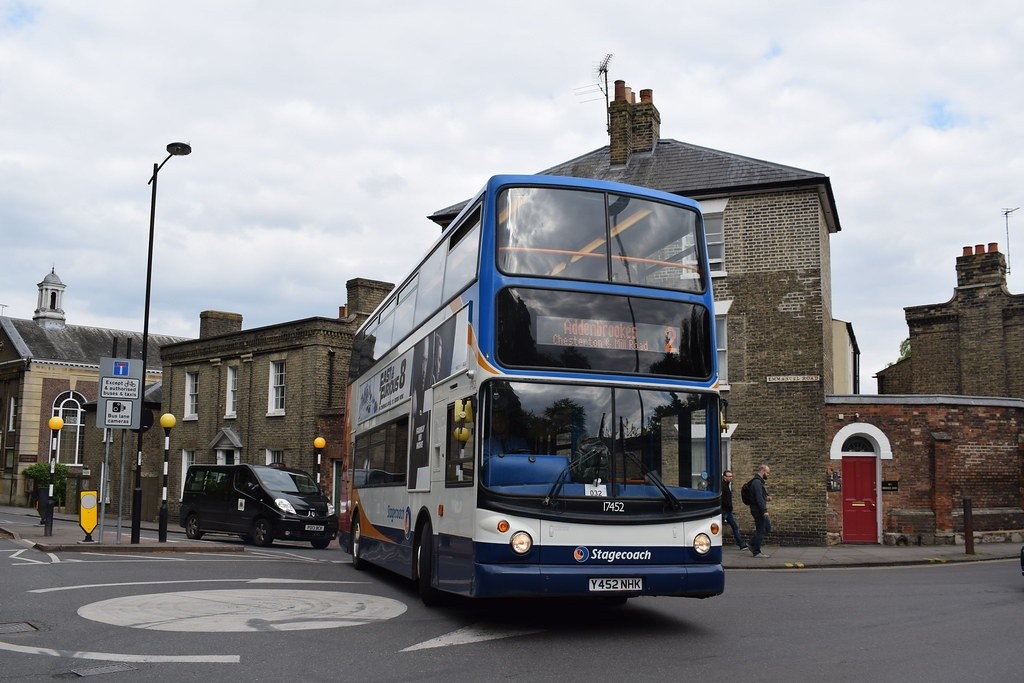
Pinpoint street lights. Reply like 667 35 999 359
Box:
313 437 326 489
131 141 191 545
159 413 177 543
43 416 64 538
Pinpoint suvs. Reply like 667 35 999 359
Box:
179 464 339 550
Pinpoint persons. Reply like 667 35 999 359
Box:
748 464 771 558
720 470 749 551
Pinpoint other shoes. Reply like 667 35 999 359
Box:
740 543 749 551
753 552 770 558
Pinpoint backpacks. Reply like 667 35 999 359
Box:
741 477 764 506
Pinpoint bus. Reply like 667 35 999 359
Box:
346 173 734 602
